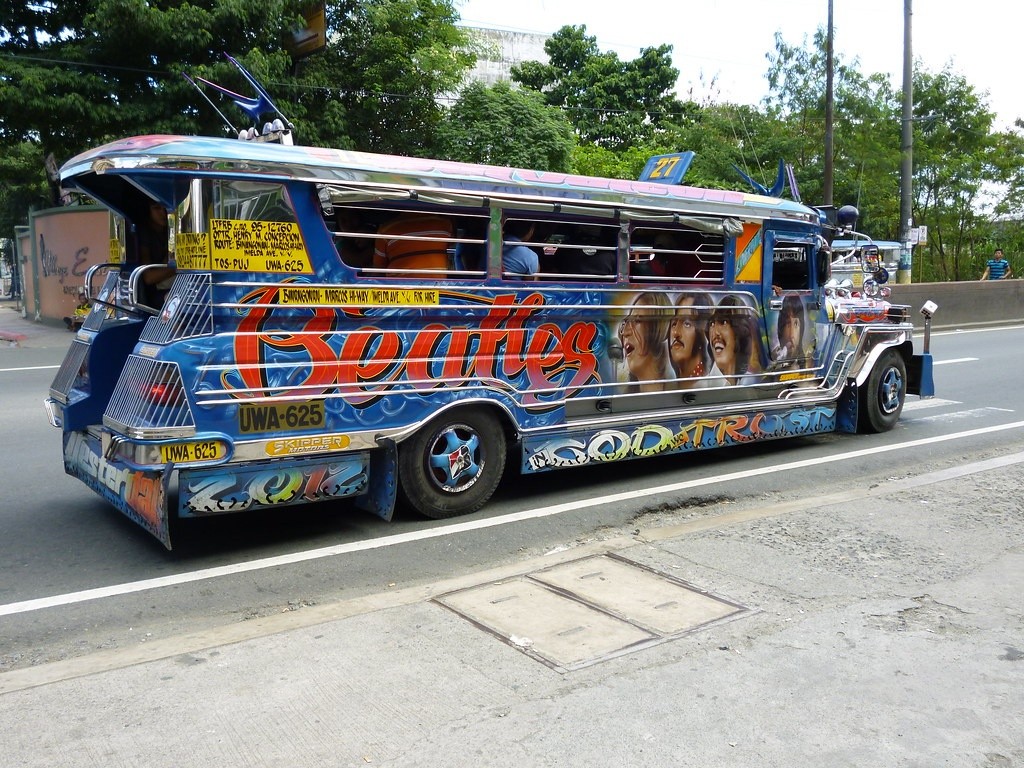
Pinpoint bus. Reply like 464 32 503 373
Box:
831 240 901 286
47 55 935 550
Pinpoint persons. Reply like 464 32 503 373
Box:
979 249 1011 280
772 285 782 296
129 199 174 304
501 216 617 282
331 210 452 281
630 234 679 276
620 289 806 391
